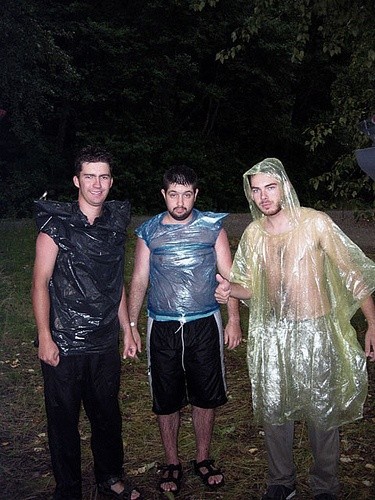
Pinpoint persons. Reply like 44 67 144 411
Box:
32 145 139 500
213 157 375 499
127 166 242 494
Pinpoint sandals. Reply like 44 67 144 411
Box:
97 475 142 500
193 459 224 489
159 462 182 496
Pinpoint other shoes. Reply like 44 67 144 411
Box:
262 484 296 500
315 493 338 500
